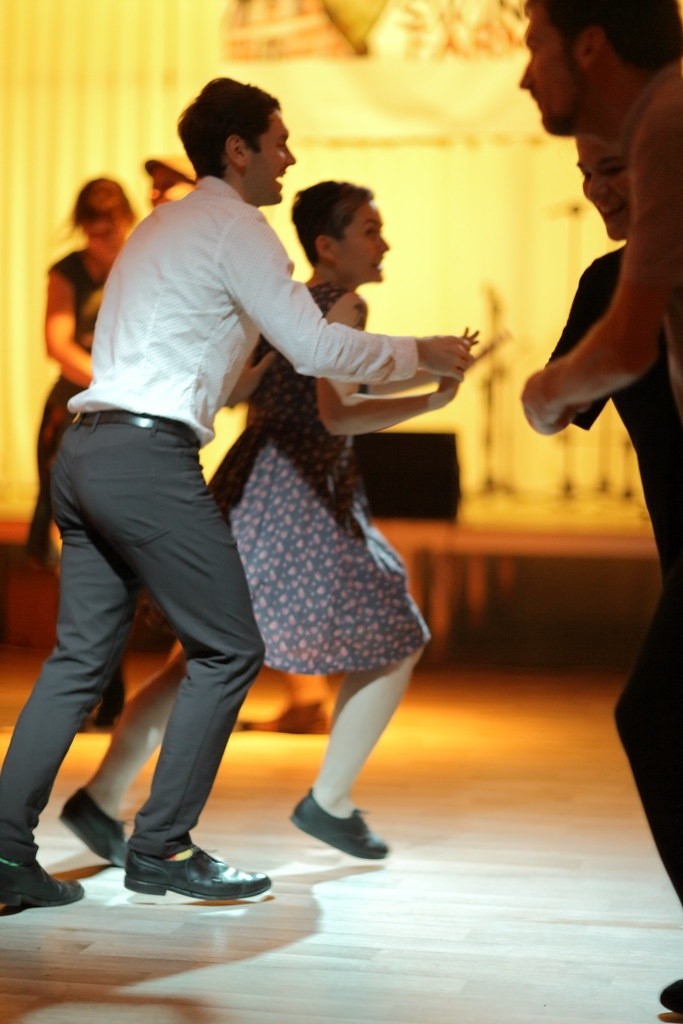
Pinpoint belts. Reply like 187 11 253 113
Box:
71 408 203 452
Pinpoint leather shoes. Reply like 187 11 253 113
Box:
0 858 83 906
125 847 271 900
60 788 128 867
290 788 387 859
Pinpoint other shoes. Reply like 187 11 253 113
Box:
660 979 683 1014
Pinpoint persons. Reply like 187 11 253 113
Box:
520 0 683 1010
0 77 472 910
61 179 480 868
30 161 200 727
